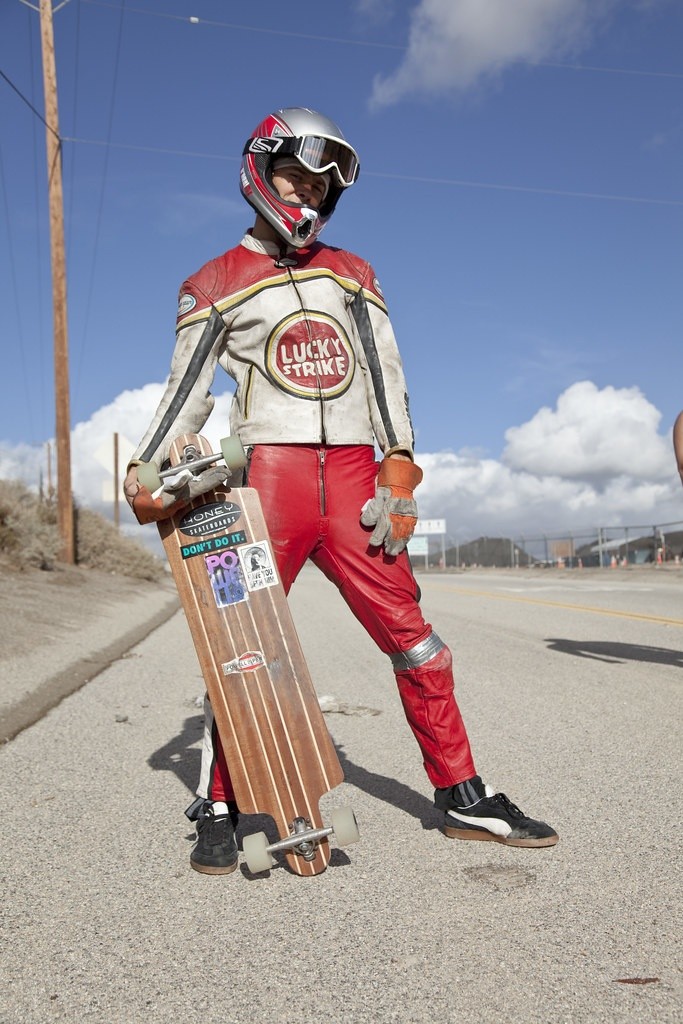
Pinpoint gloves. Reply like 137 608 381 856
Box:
131 464 233 525
360 457 423 557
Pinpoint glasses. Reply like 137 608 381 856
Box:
294 132 361 188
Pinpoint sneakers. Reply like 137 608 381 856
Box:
189 802 238 874
443 791 559 848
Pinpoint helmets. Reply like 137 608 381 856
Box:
240 107 360 250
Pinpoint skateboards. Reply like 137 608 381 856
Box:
133 433 362 877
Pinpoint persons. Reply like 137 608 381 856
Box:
123 107 559 874
